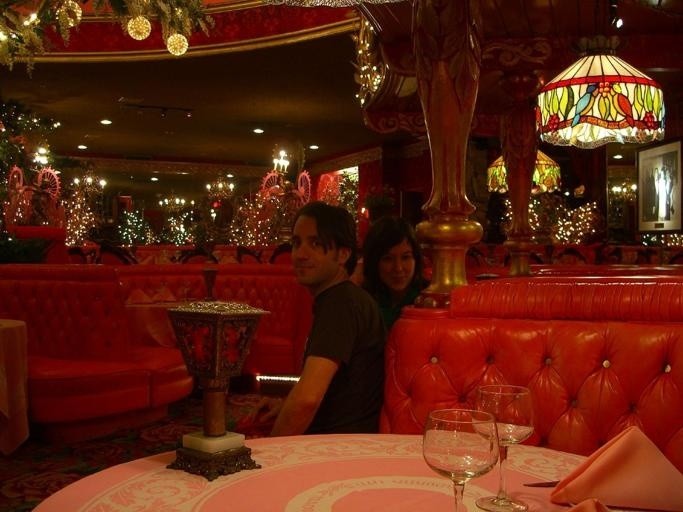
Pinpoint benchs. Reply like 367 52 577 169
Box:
0 243 315 461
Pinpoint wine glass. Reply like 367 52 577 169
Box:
424 385 535 510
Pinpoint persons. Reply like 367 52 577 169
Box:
235 200 385 435
359 219 431 320
645 167 677 220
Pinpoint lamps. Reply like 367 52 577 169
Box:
486 2 666 195
165 296 269 483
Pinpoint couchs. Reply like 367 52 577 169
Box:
375 280 680 472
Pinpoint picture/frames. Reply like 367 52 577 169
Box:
635 137 682 235
